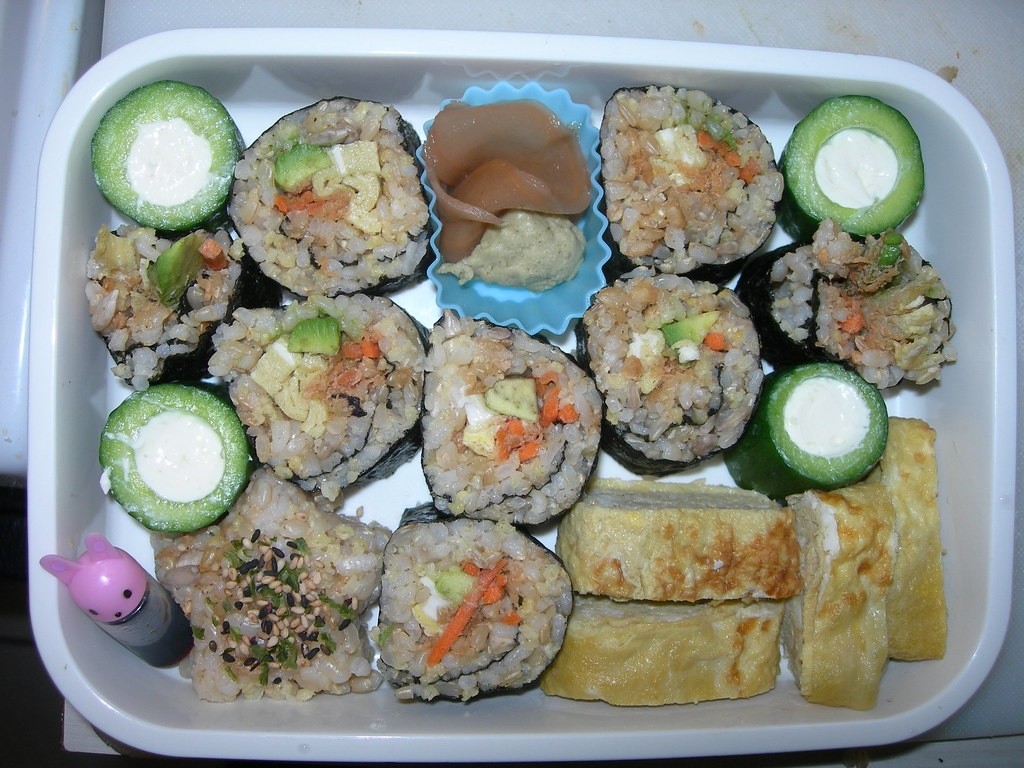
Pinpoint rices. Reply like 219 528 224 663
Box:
150 471 397 700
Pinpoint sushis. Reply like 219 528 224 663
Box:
82 81 959 702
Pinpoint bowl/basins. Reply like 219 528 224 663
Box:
28 30 1017 760
